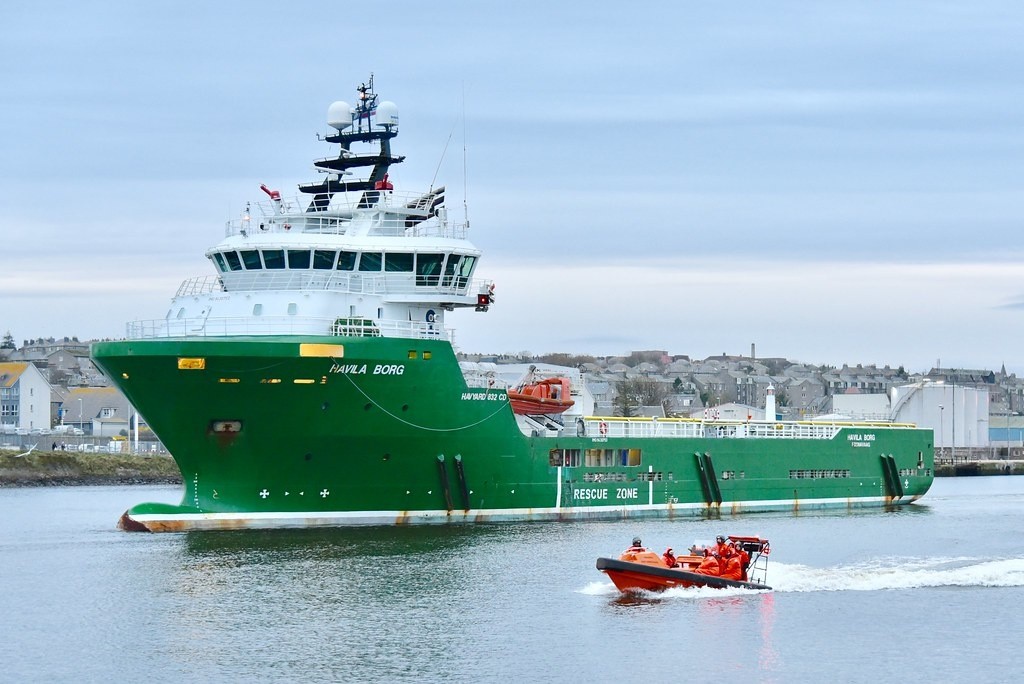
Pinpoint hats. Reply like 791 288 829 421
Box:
633 537 641 545
716 535 724 543
735 541 742 551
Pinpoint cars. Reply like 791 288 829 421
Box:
0 424 85 436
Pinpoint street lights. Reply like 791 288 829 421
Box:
938 405 944 458
78 399 82 430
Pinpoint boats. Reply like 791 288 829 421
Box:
90 73 936 534
508 377 576 414
595 535 774 597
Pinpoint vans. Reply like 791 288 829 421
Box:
57 441 129 453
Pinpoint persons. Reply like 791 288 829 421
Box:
695 535 749 582
661 546 680 568
625 537 647 553
52 441 65 451
